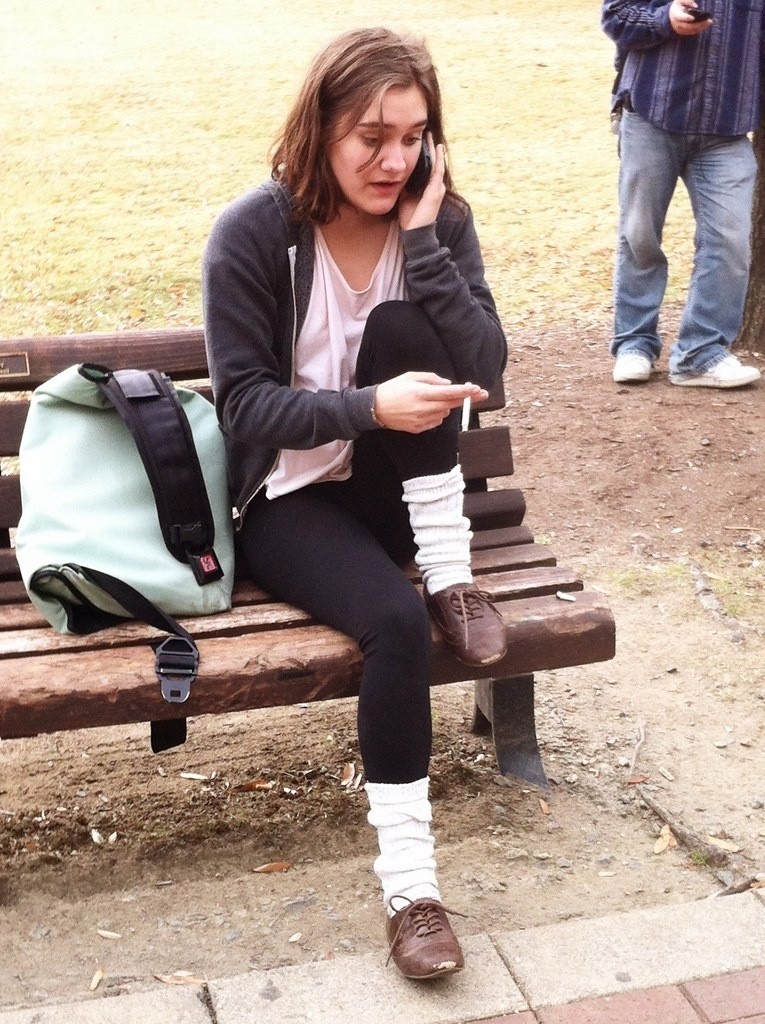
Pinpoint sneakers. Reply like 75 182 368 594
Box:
613 354 650 383
671 356 761 389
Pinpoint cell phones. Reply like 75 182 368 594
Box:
686 8 712 21
404 138 431 195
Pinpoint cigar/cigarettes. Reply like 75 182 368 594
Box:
461 381 471 433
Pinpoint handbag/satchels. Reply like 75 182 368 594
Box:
7 359 235 754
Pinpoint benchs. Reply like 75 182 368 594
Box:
0 328 619 784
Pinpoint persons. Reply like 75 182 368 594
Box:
203 26 508 979
601 0 765 388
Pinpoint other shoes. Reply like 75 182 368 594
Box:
385 894 470 981
422 573 509 668
